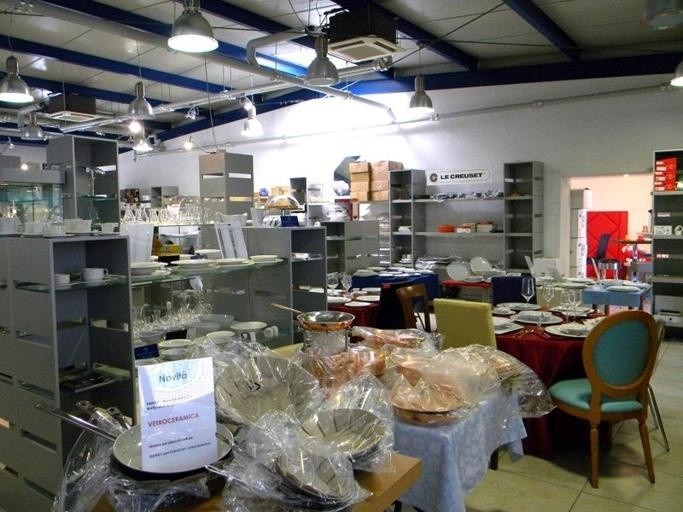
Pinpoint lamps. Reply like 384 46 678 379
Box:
126 74 157 119
168 1 217 55
126 122 144 134
0 53 35 104
304 38 342 91
240 92 266 137
186 105 198 123
406 67 436 118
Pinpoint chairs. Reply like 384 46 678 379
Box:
396 285 433 329
380 280 416 328
548 308 659 488
587 232 610 278
432 296 494 348
490 273 535 304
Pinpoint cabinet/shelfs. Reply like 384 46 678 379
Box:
0 221 326 511
648 191 682 331
321 220 379 270
387 161 544 271
0 134 118 220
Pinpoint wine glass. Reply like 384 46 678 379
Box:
520 275 581 328
326 271 352 302
121 204 210 226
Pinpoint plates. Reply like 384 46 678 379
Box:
491 302 607 339
398 259 412 264
352 266 434 278
532 277 648 292
297 286 381 308
446 256 521 284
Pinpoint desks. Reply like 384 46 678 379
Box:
326 288 378 342
351 268 436 303
105 445 421 510
372 379 527 510
613 238 651 282
444 278 651 317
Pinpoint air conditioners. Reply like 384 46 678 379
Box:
46 98 104 125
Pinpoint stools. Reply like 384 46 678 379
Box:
596 257 618 280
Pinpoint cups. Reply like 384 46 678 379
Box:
401 254 405 258
0 216 120 238
406 254 410 259
543 265 552 289
53 268 127 291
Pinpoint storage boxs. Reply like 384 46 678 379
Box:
568 237 588 266
569 210 588 238
348 160 404 201
571 187 594 210
568 263 586 278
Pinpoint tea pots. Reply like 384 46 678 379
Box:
589 255 619 289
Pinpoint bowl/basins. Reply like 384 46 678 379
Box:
130 249 283 281
213 349 391 512
394 358 430 386
292 253 308 259
391 382 464 426
132 286 268 370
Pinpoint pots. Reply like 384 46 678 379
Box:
112 421 234 507
